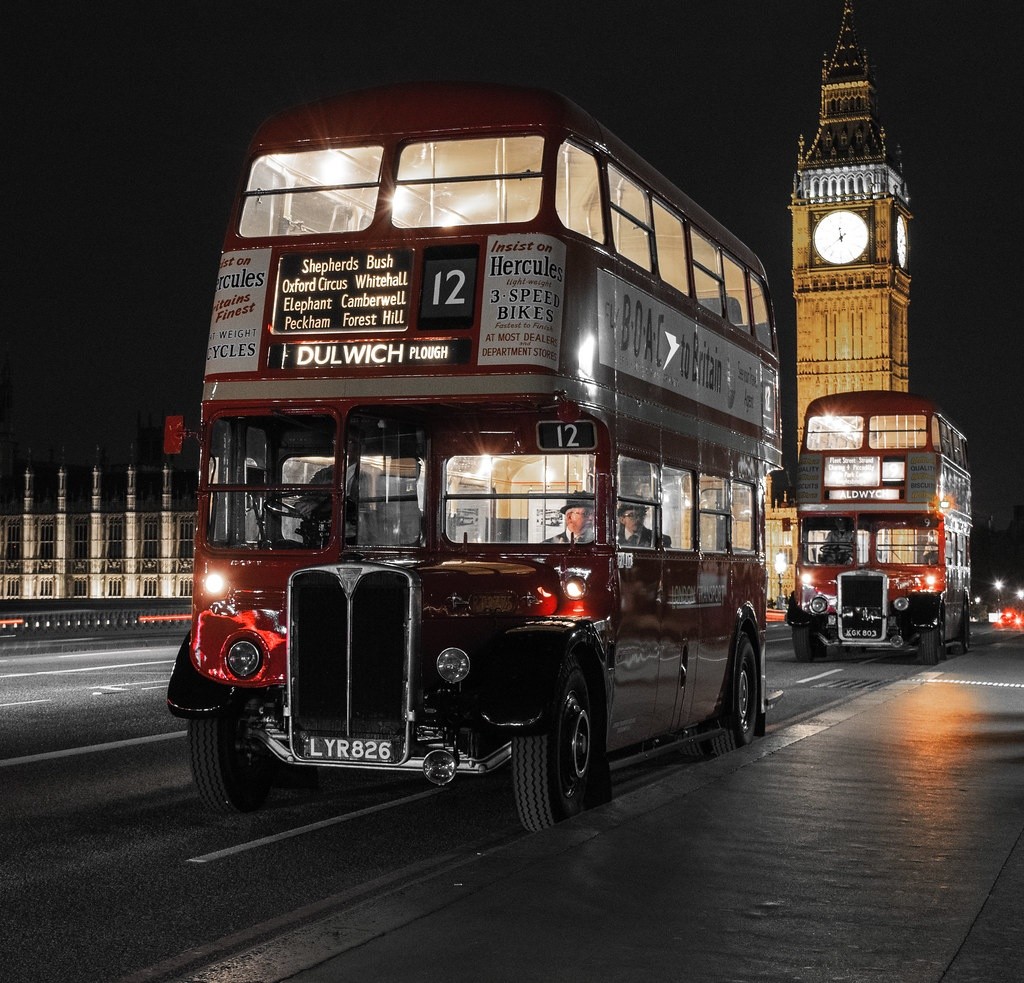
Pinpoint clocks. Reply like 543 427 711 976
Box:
897 214 907 268
811 209 868 265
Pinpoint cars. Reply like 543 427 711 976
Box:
992 612 1022 631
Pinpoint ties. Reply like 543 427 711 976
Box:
630 534 639 546
577 537 583 542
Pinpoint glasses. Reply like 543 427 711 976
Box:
623 512 647 519
331 439 353 445
570 511 596 516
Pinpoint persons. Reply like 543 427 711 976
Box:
294 429 377 522
543 491 593 543
922 540 938 563
618 494 670 547
820 516 855 563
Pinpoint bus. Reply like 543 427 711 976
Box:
786 390 972 665
163 85 778 833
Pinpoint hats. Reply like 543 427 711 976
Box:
560 492 594 514
616 494 649 517
